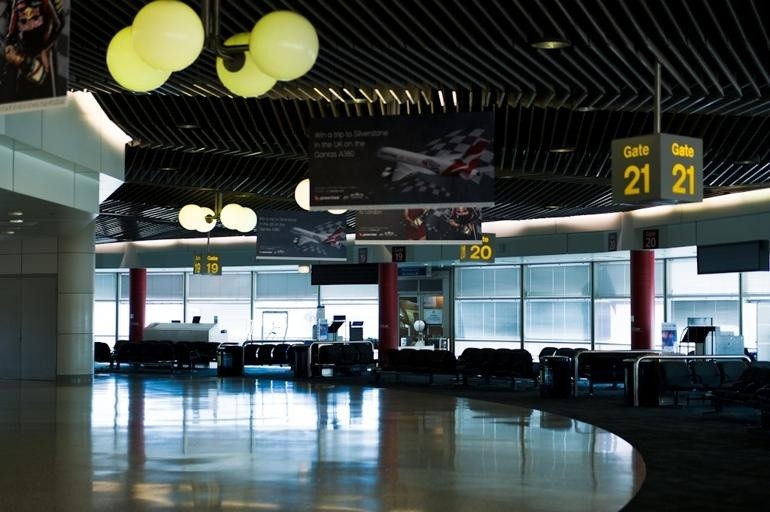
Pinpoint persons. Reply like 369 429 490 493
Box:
405 208 429 240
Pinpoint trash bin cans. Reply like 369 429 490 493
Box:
291 344 312 380
217 345 243 378
540 356 572 397
623 358 658 406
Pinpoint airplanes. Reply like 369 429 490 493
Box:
290 224 344 254
376 128 490 204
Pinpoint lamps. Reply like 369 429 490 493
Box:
297 265 310 275
530 39 573 51
168 185 260 237
103 0 320 106
